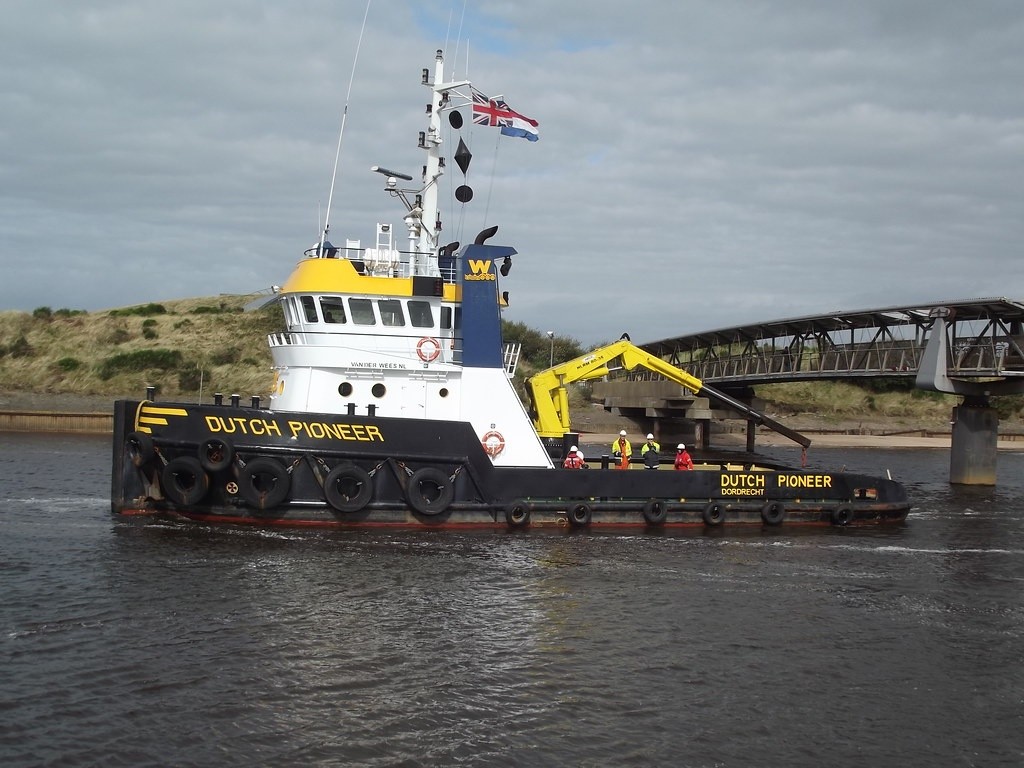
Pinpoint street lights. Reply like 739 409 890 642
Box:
547 332 554 368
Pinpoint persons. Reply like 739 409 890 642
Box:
783 346 792 372
673 443 694 502
612 430 632 469
563 446 591 469
641 434 660 469
719 462 751 471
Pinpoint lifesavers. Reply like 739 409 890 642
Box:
198 434 237 473
404 468 456 519
567 500 592 528
322 464 373 514
128 431 157 469
239 456 292 514
482 431 505 456
505 500 530 526
643 499 668 525
760 500 786 526
161 457 209 510
833 504 856 526
416 337 440 363
703 502 726 526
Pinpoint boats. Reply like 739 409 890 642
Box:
109 0 915 535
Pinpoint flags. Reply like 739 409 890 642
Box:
468 85 539 143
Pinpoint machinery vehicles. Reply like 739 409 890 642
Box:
524 332 811 469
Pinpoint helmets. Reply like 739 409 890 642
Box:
676 444 686 449
646 433 654 440
570 445 578 451
619 430 627 435
